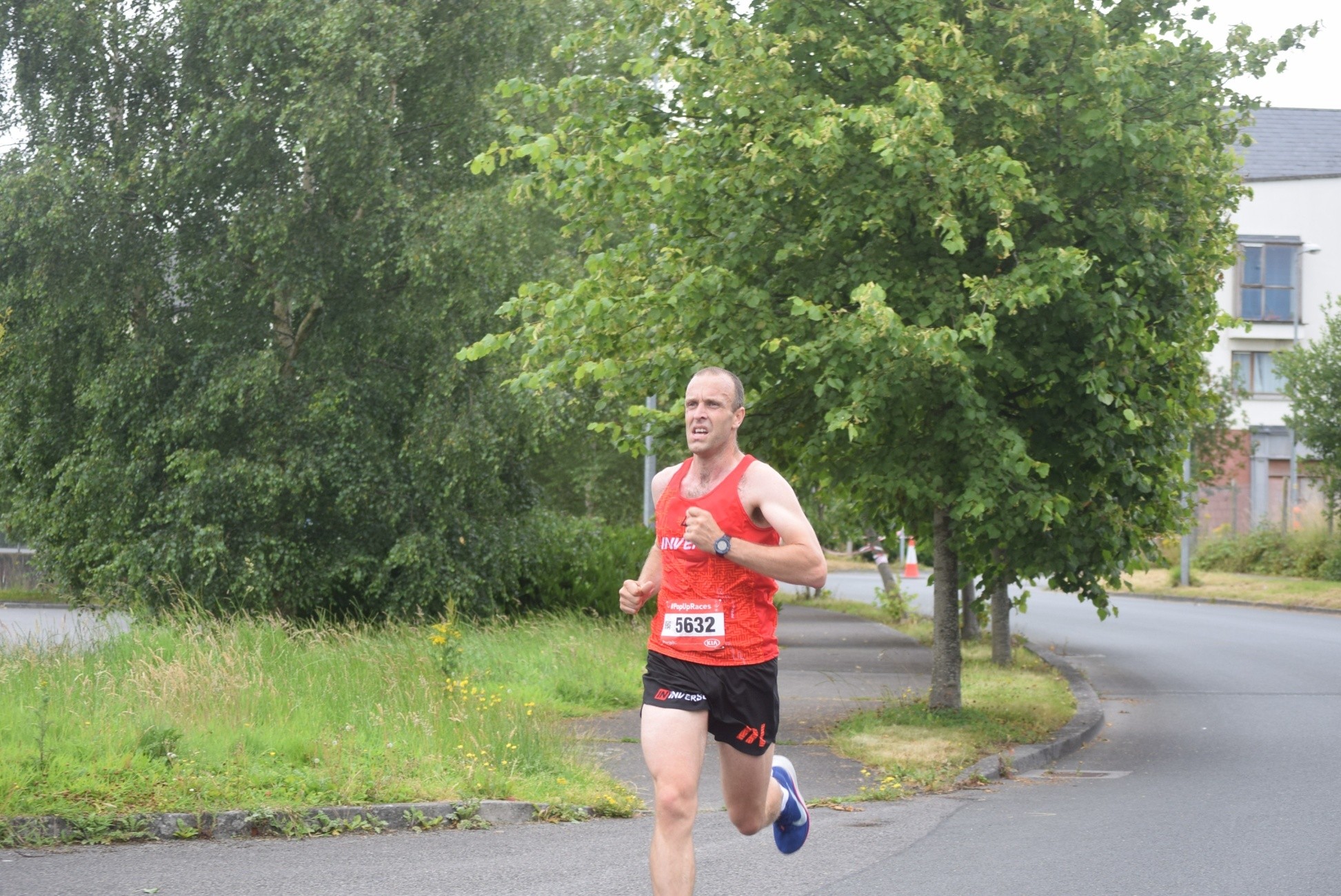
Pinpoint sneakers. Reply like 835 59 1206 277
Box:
769 754 810 856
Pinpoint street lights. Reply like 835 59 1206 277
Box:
1290 242 1323 536
1180 239 1269 590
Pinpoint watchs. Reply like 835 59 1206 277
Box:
714 534 731 558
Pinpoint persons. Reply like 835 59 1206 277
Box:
619 367 827 896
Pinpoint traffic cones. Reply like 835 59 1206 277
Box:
898 536 927 580
